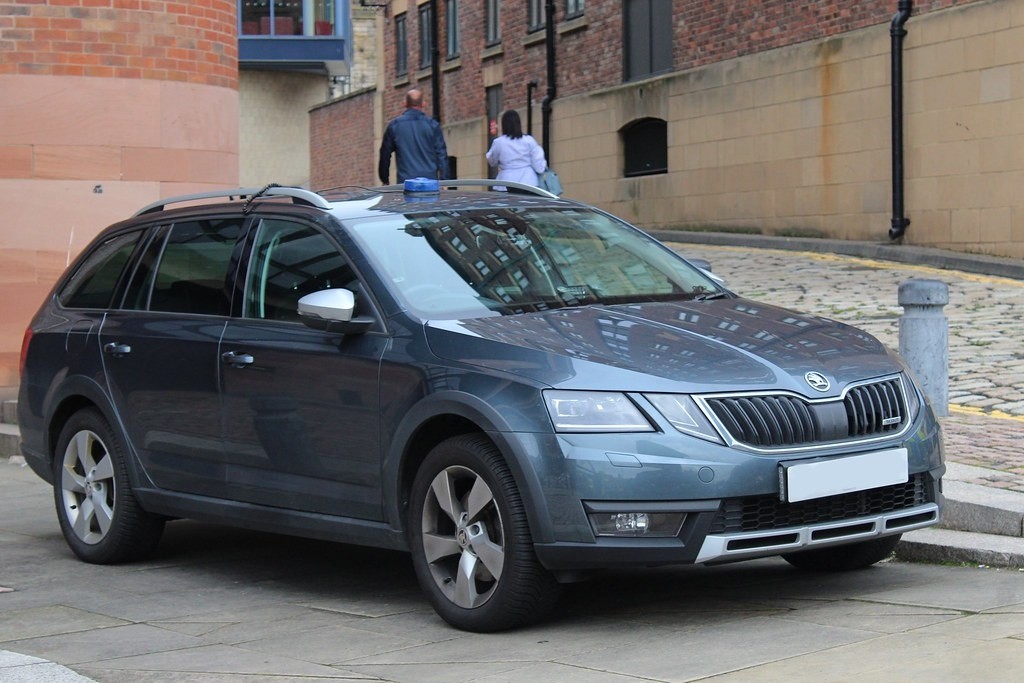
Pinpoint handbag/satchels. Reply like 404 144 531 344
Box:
536 165 563 195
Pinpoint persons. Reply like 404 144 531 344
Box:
379 89 452 192
485 110 549 194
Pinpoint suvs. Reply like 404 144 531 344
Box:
15 179 944 636
